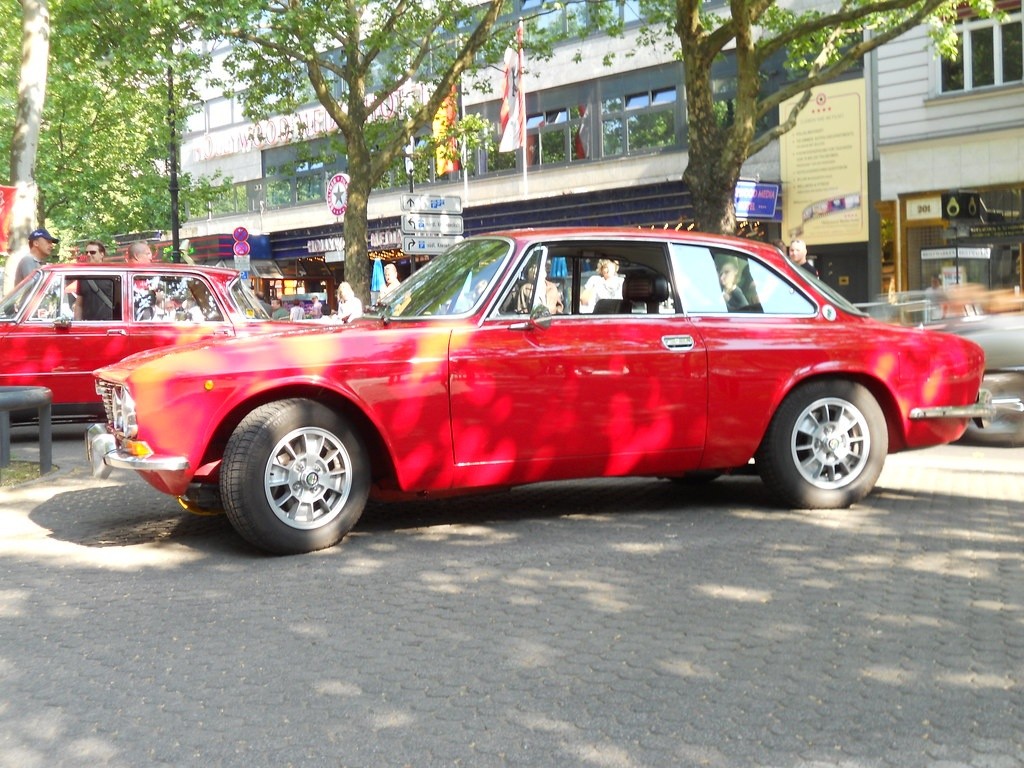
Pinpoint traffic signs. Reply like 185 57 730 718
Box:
402 234 465 255
401 214 464 235
400 193 463 214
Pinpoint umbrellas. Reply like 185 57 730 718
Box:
371 255 386 292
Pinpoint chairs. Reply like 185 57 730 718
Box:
621 271 671 312
593 299 629 314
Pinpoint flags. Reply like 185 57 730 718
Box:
497 30 523 153
432 82 464 175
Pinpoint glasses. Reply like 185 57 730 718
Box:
85 251 100 255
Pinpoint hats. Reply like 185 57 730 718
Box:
28 229 59 243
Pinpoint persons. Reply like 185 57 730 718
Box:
473 262 564 316
15 229 60 318
128 242 165 320
337 282 363 322
270 297 290 320
584 259 624 312
376 264 401 302
308 295 323 318
73 242 113 321
319 304 331 320
289 299 306 321
719 240 817 310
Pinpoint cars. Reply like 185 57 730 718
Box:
0 263 335 429
84 225 985 555
